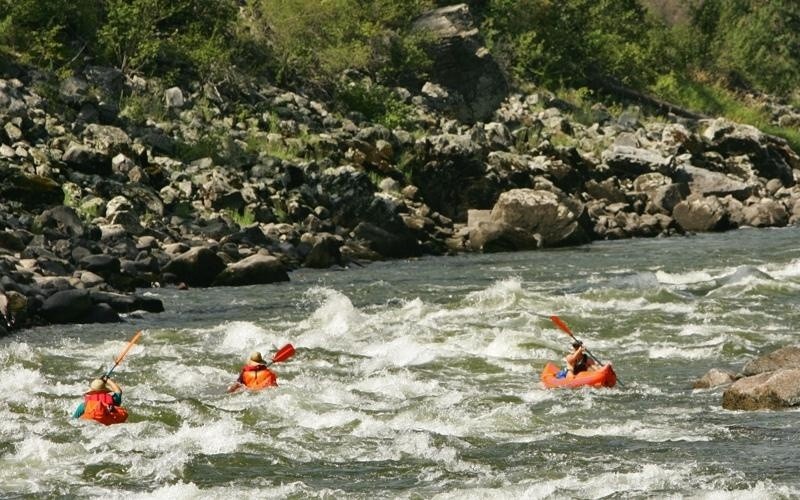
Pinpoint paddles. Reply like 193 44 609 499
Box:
105 331 143 376
265 343 296 369
550 316 626 388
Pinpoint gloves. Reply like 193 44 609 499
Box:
100 375 110 384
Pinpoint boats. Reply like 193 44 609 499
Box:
540 361 617 390
80 400 128 427
246 370 277 389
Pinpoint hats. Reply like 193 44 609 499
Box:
86 379 112 392
245 351 269 367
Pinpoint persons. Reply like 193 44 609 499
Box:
565 337 594 377
73 376 123 420
237 353 277 389
